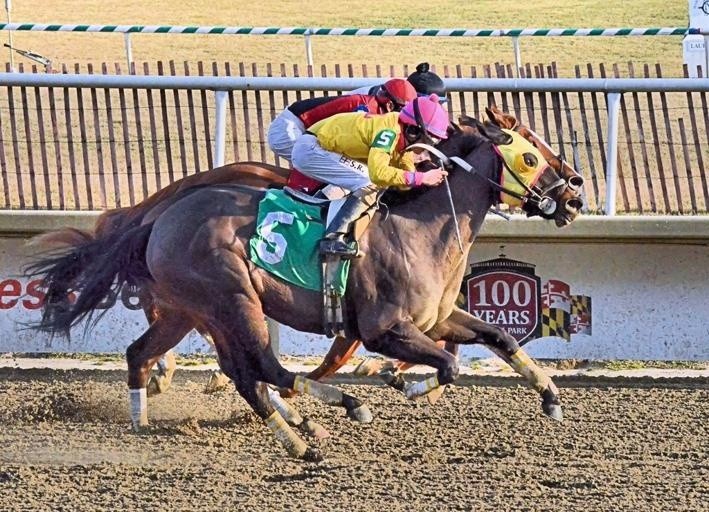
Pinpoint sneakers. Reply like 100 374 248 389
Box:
316 232 365 263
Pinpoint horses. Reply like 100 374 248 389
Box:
8 106 583 463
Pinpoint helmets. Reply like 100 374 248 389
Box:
376 77 417 106
405 63 447 103
399 94 449 140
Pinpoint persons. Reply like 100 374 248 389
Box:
268 62 453 259
